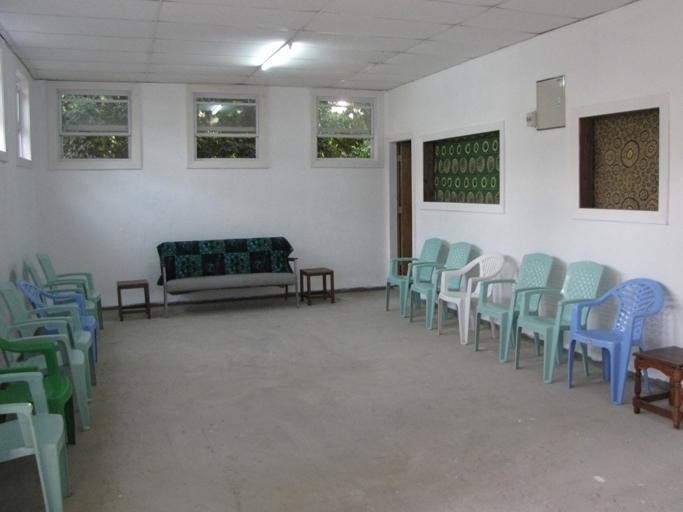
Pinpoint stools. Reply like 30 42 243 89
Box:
117 279 150 321
300 267 335 305
632 346 683 429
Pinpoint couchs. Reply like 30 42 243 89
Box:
157 237 300 318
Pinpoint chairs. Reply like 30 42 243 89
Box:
0 253 103 512
